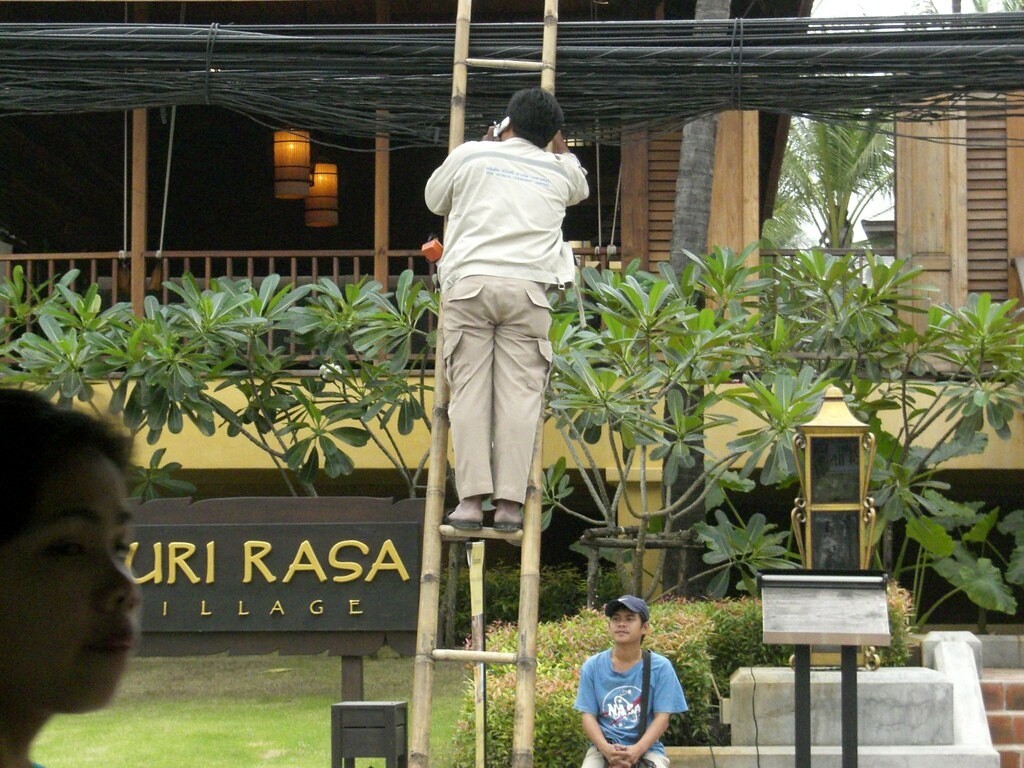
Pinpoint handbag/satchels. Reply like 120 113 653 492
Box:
603 756 656 768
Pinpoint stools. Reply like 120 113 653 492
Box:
331 701 408 767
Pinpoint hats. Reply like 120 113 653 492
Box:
605 595 649 622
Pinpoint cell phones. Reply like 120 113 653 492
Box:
493 116 510 138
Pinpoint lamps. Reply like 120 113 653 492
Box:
271 124 311 200
303 161 339 227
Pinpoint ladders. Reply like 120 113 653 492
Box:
404 0 559 768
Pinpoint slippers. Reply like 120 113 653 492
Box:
494 521 523 532
443 508 482 530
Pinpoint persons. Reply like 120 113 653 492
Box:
424 87 593 530
0 387 141 768
571 594 689 768
